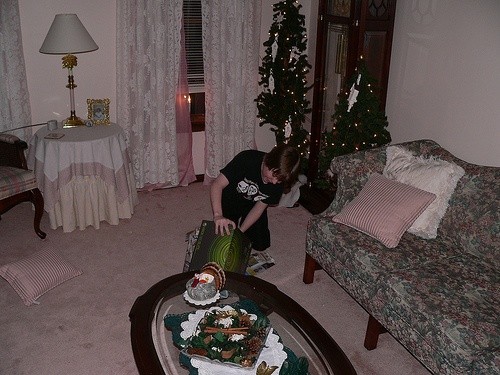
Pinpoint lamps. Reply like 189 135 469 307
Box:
39 14 99 129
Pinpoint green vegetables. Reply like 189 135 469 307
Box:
191 309 269 367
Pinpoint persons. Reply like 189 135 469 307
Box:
95 108 104 119
209 143 303 252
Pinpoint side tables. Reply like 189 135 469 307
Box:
26 123 140 234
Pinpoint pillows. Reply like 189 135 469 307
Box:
383 145 466 239
0 247 84 305
333 172 437 249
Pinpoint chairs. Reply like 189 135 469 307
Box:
0 133 47 239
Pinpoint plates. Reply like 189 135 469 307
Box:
180 311 272 369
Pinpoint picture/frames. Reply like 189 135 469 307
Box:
87 98 111 126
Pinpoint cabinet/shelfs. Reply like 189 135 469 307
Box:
298 0 397 215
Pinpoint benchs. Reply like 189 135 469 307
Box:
302 140 500 375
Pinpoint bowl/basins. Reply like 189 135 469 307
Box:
187 277 217 301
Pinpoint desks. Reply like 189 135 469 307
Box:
128 272 356 375
